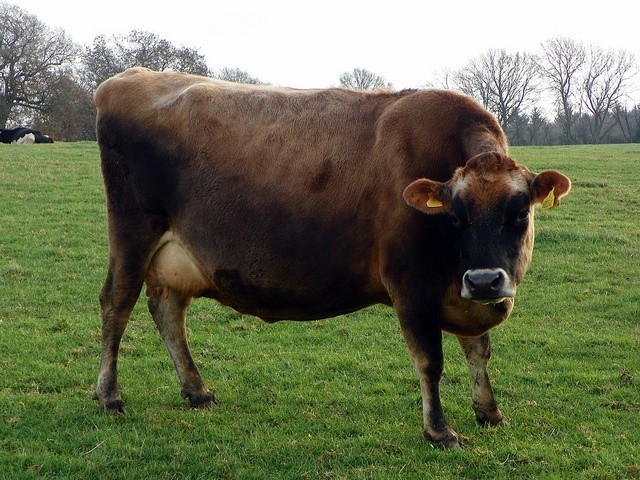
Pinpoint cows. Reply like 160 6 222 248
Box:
89 63 574 453
